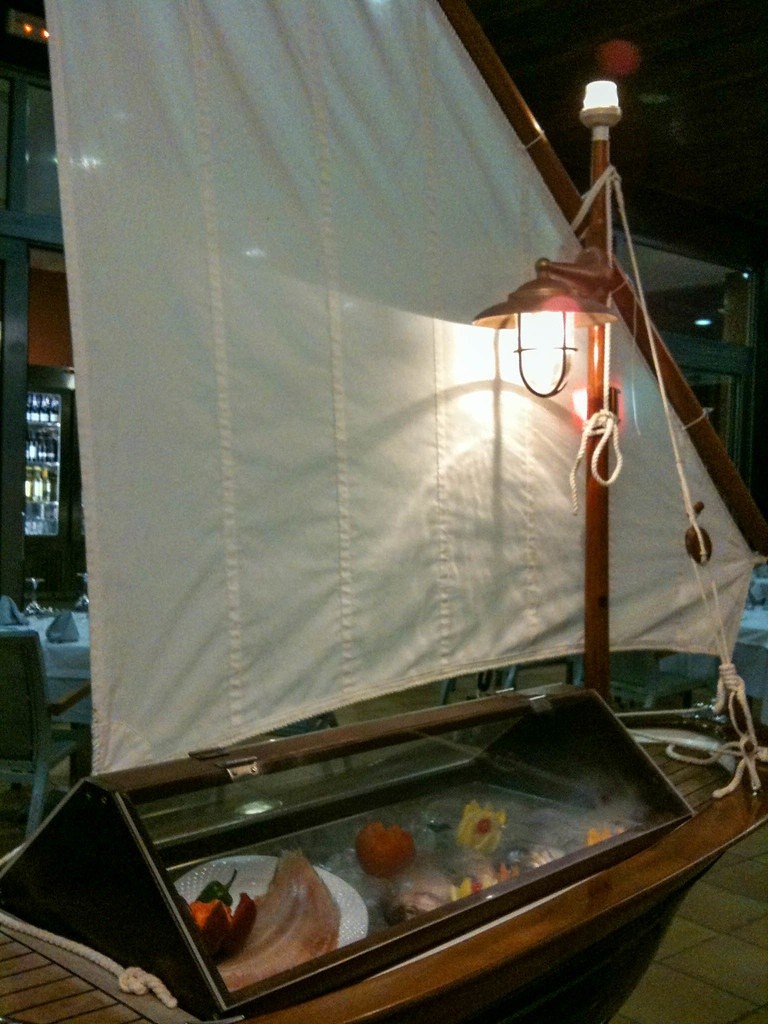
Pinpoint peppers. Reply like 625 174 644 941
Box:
196 868 237 906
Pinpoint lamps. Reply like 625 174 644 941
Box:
473 250 624 399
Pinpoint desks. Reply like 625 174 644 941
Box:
5 614 92 782
608 569 768 742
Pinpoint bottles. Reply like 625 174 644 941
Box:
25 430 55 460
24 466 51 502
24 503 56 535
26 392 59 421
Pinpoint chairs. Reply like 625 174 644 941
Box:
1 625 93 840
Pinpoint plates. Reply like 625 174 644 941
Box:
172 855 369 950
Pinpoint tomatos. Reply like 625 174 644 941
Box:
478 818 490 833
357 821 414 875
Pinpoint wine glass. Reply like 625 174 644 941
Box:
24 577 46 629
73 572 89 625
